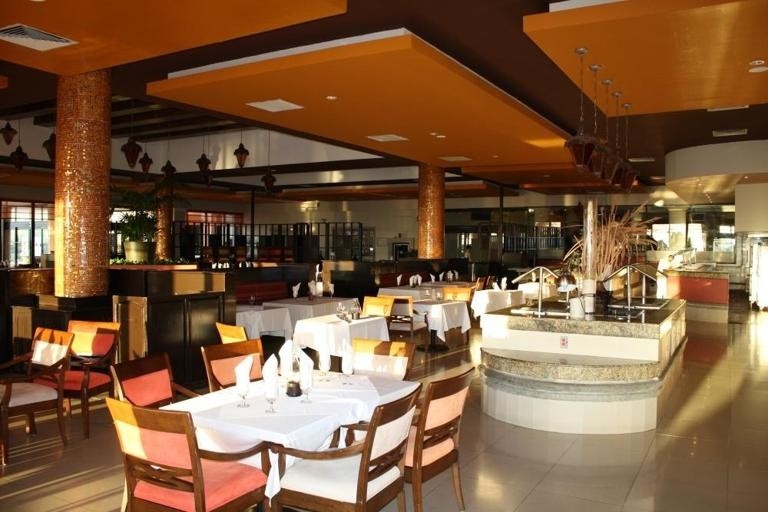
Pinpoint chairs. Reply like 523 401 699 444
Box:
471 277 485 291
34 320 122 439
486 276 495 289
352 338 416 381
270 382 423 512
403 367 475 512
361 296 395 318
442 287 475 345
110 352 201 408
0 327 75 466
379 294 429 353
201 338 264 393
105 397 269 512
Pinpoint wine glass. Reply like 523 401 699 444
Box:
293 291 298 301
300 375 313 403
279 359 292 381
329 288 334 299
319 356 331 384
264 384 278 413
341 356 351 385
237 379 250 406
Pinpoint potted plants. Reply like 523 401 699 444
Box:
108 187 159 263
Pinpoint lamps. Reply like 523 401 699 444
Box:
612 91 623 158
260 129 276 194
601 80 623 186
195 134 210 174
234 127 250 169
621 102 641 194
41 126 57 160
618 182 622 184
1 119 16 145
119 136 143 169
588 64 608 180
10 120 28 171
160 140 175 180
138 141 152 176
564 46 595 170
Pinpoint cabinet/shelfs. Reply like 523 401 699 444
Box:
112 290 226 400
